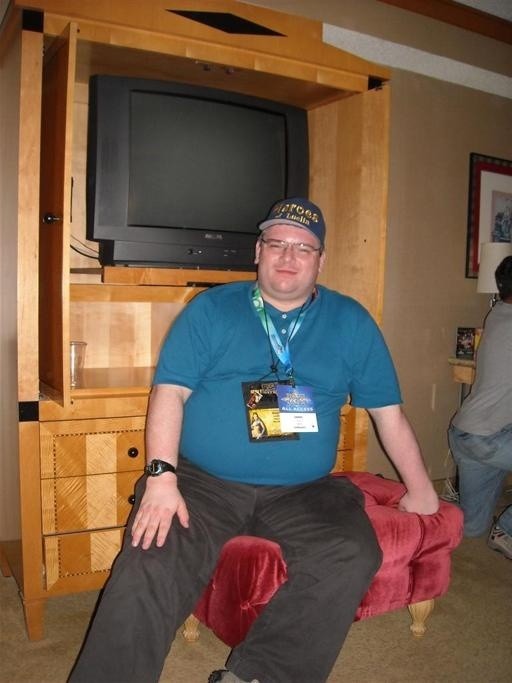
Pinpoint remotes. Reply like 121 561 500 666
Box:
186 280 226 288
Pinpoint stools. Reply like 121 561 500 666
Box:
179 470 464 643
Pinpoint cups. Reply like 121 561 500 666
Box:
70 341 88 386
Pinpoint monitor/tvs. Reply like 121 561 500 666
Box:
84 74 311 270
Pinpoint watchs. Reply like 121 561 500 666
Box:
143 459 177 478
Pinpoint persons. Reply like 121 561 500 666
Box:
445 254 512 562
66 197 442 683
250 411 265 440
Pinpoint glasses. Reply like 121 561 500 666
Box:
259 236 321 256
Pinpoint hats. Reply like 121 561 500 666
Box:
256 194 326 247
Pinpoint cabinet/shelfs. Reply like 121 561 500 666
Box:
0 0 390 641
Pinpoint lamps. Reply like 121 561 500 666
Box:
476 242 510 306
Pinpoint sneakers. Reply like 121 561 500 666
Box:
487 516 512 561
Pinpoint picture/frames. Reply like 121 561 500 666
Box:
463 153 511 278
